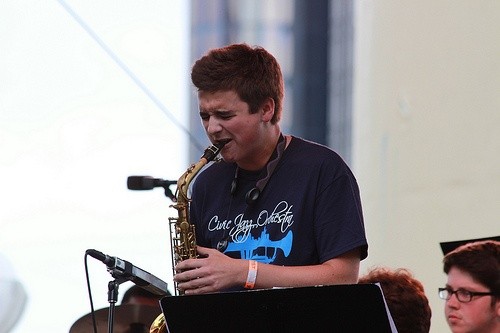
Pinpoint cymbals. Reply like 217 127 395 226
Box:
68 304 163 333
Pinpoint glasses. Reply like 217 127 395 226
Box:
438 288 500 303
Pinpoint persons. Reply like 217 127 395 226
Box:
358 266 432 333
437 241 500 333
173 42 369 295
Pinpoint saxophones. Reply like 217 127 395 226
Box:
149 140 230 333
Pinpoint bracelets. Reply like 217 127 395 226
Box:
244 260 257 289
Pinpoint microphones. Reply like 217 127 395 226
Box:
127 175 178 190
87 249 168 297
